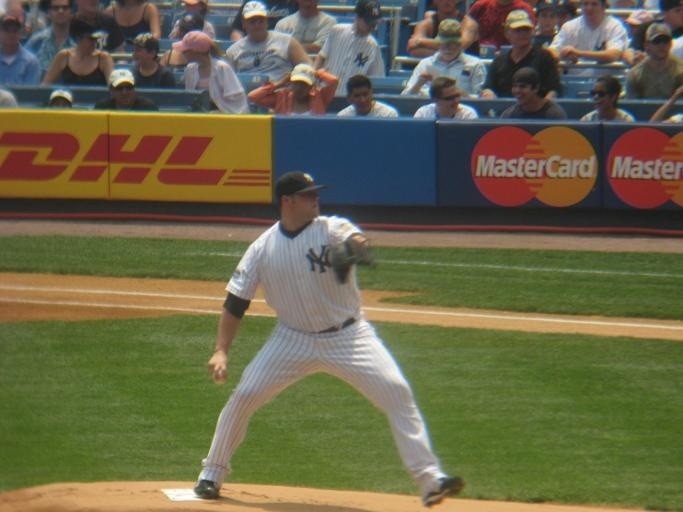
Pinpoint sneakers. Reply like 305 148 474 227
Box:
194 480 220 498
421 475 465 509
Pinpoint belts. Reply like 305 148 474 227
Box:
319 316 356 332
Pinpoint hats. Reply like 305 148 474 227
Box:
512 67 546 91
272 171 329 200
354 0 384 26
109 68 136 87
1 16 22 27
435 19 462 45
172 31 213 54
71 15 104 39
290 63 317 85
535 3 559 18
505 9 534 30
644 22 672 41
126 33 160 52
242 1 267 19
625 9 652 26
48 89 73 104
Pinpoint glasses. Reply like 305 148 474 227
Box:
116 85 133 91
651 37 671 45
50 5 71 11
590 89 609 97
439 92 461 101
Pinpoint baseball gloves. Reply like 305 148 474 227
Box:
332 238 378 285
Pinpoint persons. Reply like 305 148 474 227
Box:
191 170 464 509
1 0 683 124
